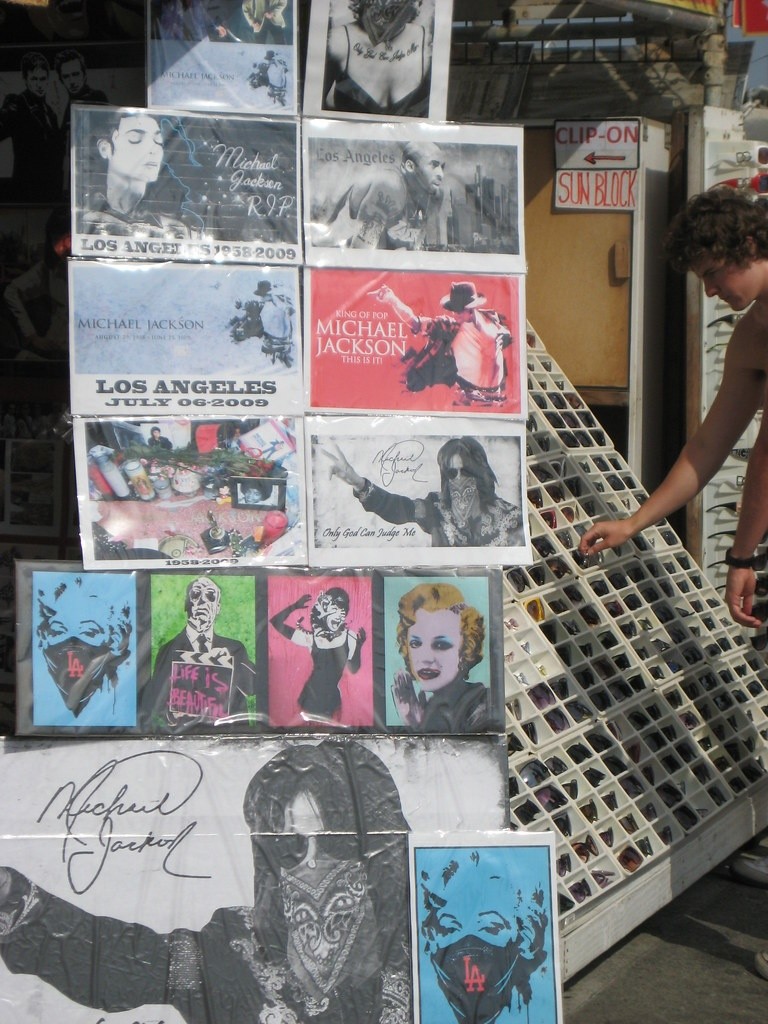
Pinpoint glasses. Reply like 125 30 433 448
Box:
591 870 615 886
489 311 768 798
599 827 614 848
514 800 540 826
533 786 568 813
558 893 575 914
578 800 598 825
554 813 573 839
568 879 592 904
572 835 599 863
601 790 621 812
641 802 658 822
695 808 709 818
617 846 643 873
656 826 672 845
674 805 697 830
618 813 641 834
656 783 686 808
553 853 572 877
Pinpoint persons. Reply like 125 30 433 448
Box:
578 185 767 978
319 433 524 546
375 281 513 406
311 142 447 252
78 113 191 241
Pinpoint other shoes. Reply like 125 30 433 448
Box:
754 949 768 980
727 833 768 884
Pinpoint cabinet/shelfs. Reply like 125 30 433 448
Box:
500 317 768 990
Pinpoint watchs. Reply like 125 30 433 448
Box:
725 546 755 569
